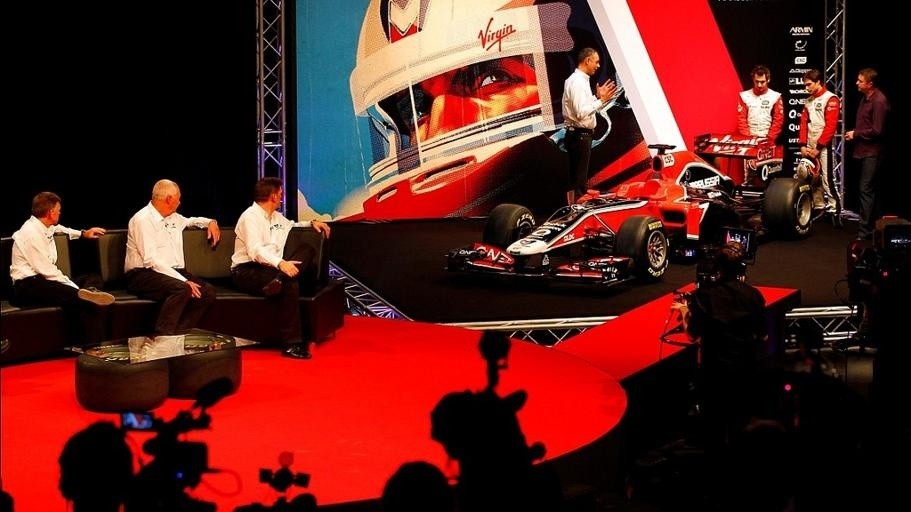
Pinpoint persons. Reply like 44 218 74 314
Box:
561 47 618 205
737 66 785 143
59 420 217 512
845 66 888 239
230 176 332 361
792 68 843 218
348 0 602 220
6 190 117 355
119 178 220 335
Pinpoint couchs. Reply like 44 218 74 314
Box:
0 226 346 369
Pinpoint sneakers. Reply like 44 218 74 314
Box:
77 287 118 306
811 209 839 225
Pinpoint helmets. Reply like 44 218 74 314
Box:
347 0 550 220
796 154 822 183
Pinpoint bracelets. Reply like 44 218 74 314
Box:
310 220 316 228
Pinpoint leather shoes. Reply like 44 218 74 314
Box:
262 278 285 297
281 342 312 359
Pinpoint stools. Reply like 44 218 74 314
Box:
73 334 242 421
